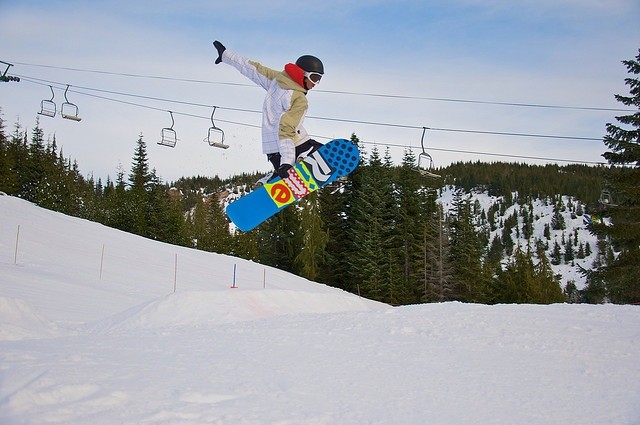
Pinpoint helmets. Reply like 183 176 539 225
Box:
295 55 325 74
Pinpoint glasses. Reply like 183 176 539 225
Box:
303 71 324 85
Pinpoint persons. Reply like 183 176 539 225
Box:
213 41 324 190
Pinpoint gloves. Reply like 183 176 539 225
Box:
213 40 226 64
274 164 292 178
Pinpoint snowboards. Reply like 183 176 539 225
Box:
226 139 359 232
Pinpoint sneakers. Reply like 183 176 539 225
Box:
254 172 274 187
296 146 315 162
